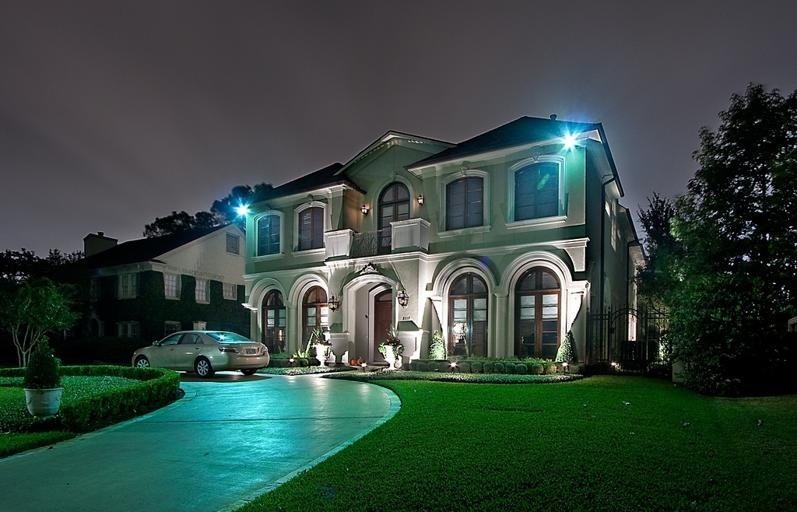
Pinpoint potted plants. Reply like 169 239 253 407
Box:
377 327 403 371
311 328 332 367
21 335 65 419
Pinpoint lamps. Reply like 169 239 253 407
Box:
359 204 369 217
557 357 571 375
416 194 424 206
327 296 339 313
287 358 296 367
446 360 461 375
397 291 409 309
358 361 369 372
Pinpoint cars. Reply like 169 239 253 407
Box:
132 330 269 378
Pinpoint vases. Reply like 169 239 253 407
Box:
328 333 349 367
